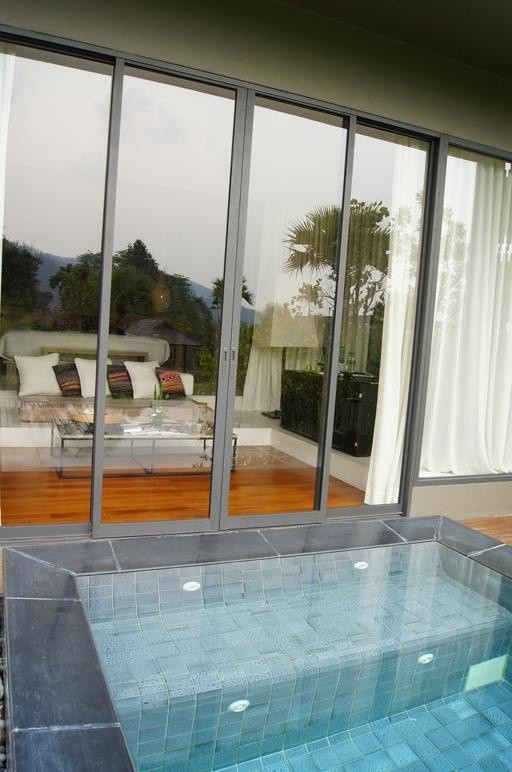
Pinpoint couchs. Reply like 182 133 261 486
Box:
0 331 216 426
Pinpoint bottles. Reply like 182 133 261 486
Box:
346 351 357 373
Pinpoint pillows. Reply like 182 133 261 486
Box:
12 353 187 400
